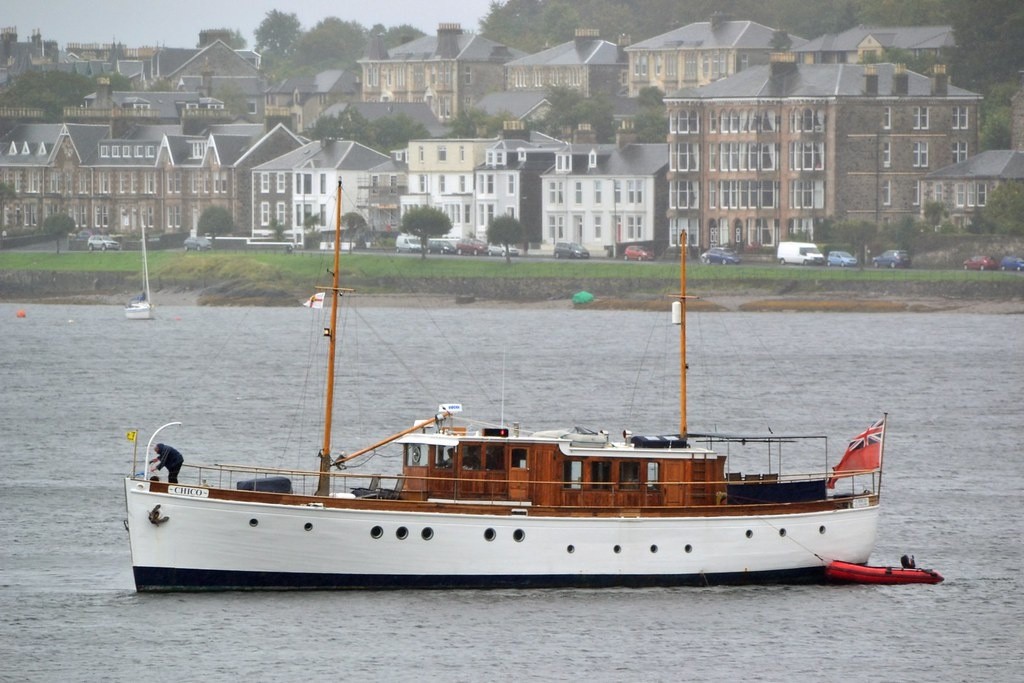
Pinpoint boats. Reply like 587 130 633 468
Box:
827 560 945 585
122 176 887 594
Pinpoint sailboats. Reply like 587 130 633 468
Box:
123 210 155 320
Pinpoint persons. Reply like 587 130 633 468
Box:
436 448 454 469
150 444 184 483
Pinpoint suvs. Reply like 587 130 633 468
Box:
872 249 911 268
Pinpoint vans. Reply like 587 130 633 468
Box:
395 234 426 253
776 241 825 268
553 242 590 259
428 240 455 255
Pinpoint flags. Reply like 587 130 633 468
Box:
302 291 325 309
825 417 884 489
125 429 137 442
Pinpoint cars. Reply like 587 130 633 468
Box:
456 237 488 256
624 245 655 261
826 250 857 268
999 256 1024 273
87 235 120 251
963 255 999 271
701 246 743 264
183 237 212 251
487 242 520 258
